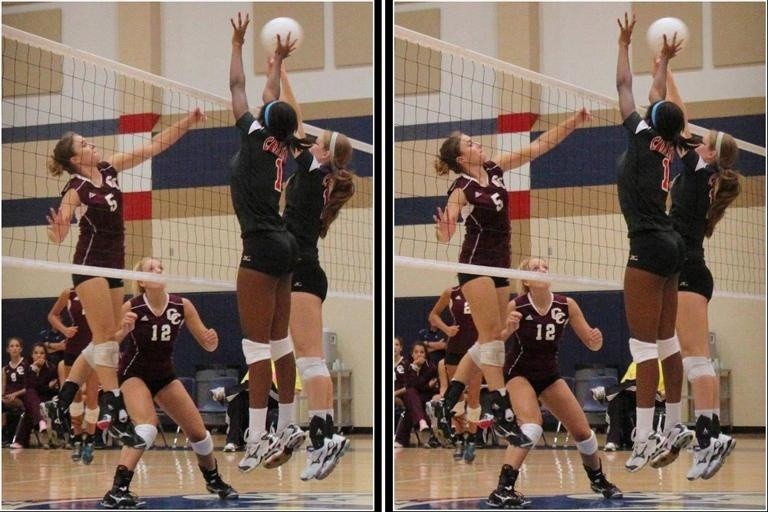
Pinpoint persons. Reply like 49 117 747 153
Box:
47 286 99 463
431 107 593 447
228 13 308 472
209 359 302 452
47 106 208 446
429 283 482 462
1 314 108 449
651 56 741 478
102 255 239 508
393 310 491 451
263 56 354 480
485 258 623 506
614 12 695 471
590 358 665 452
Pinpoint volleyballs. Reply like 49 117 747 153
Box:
262 17 303 52
647 16 688 56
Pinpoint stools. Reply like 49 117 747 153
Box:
625 405 665 450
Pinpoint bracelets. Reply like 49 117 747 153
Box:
234 45 241 50
619 45 627 48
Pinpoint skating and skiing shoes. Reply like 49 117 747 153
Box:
485 489 524 507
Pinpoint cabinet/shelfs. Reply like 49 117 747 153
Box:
291 370 353 437
678 368 734 438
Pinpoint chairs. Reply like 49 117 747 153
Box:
537 377 573 450
184 377 240 449
152 377 195 450
560 376 621 448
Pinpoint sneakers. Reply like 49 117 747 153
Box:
206 480 239 501
11 397 147 466
236 423 354 481
589 381 621 457
648 422 697 470
624 427 667 473
207 384 226 404
396 420 489 464
683 430 739 483
221 441 238 454
588 480 625 500
100 490 149 508
491 419 532 450
